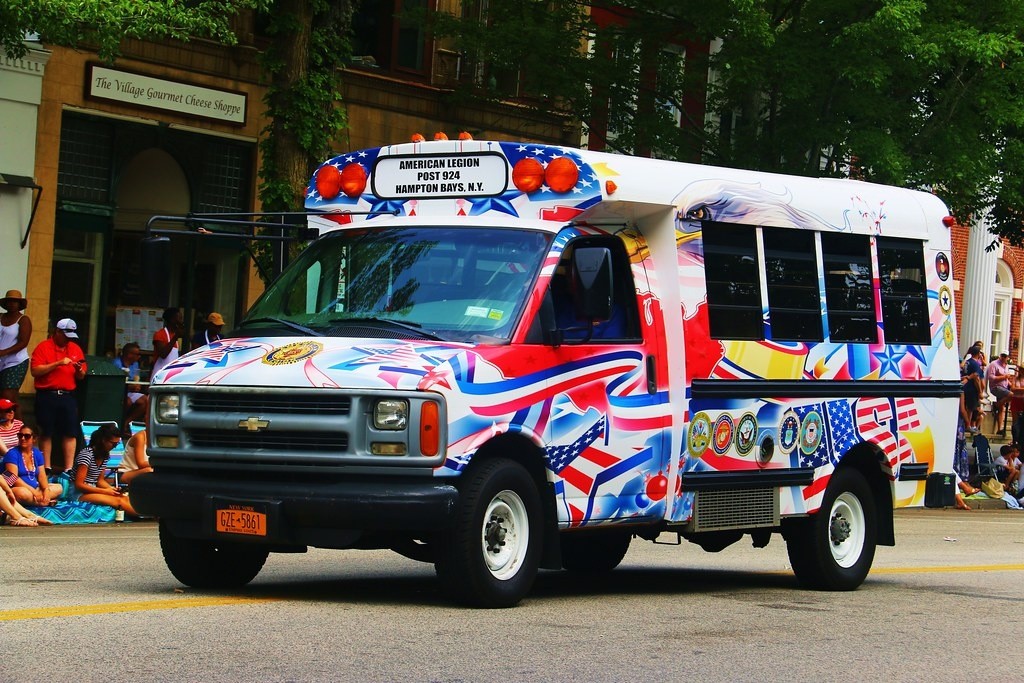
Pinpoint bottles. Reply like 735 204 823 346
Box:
115 505 124 522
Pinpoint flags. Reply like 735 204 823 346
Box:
2 469 18 487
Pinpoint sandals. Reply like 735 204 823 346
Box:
30 516 53 526
9 516 38 527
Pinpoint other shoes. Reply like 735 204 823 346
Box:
44 466 55 476
62 469 76 482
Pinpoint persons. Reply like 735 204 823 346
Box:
956 341 1024 511
115 427 154 483
0 398 63 527
0 290 32 402
70 423 142 518
149 307 185 381
112 342 148 438
191 313 225 350
30 318 88 477
510 252 627 341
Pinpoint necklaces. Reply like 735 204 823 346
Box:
22 451 36 481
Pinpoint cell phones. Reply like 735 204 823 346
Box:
78 360 85 364
117 487 129 493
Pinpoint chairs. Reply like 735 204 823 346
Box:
79 421 130 492
128 421 147 436
972 435 999 481
391 283 478 311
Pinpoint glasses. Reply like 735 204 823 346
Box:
108 440 119 448
129 352 141 359
61 329 78 333
17 432 31 439
7 405 16 413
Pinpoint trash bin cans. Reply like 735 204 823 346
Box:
73 355 129 471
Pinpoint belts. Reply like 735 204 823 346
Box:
49 390 70 395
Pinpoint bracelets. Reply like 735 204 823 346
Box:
966 377 969 381
79 368 82 372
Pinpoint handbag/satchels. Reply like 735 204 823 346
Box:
19 358 36 394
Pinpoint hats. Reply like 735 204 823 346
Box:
0 398 16 409
0 290 27 311
1000 350 1010 356
972 345 983 353
207 312 224 326
56 318 79 339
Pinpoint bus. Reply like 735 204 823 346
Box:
143 133 965 610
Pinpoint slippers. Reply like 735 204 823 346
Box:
956 504 971 510
965 487 981 497
943 536 957 540
